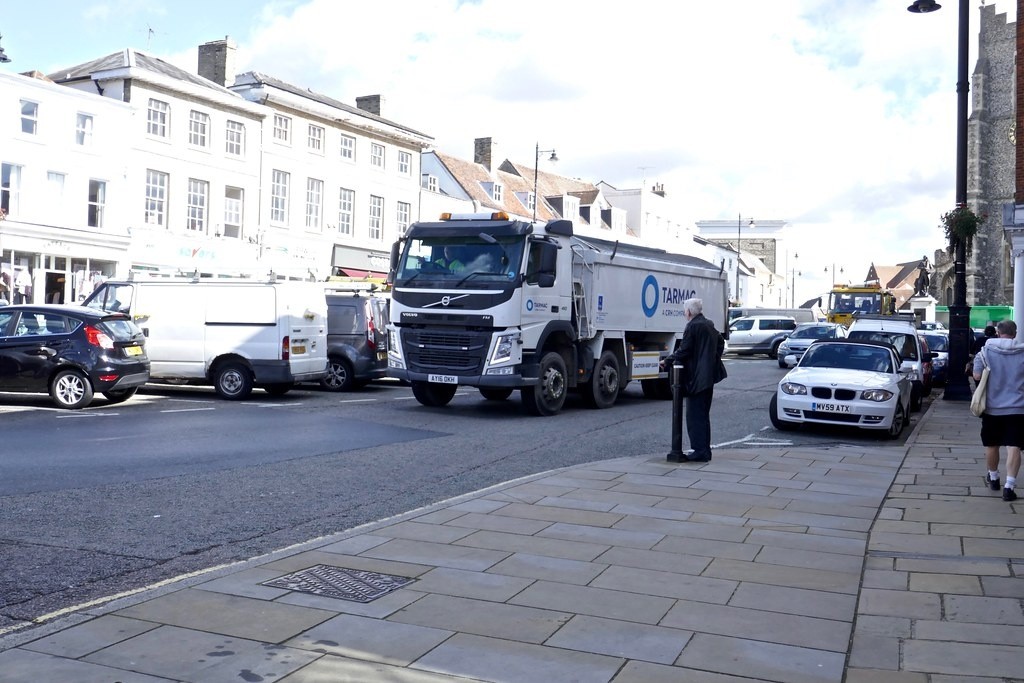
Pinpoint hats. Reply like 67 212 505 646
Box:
985 326 996 337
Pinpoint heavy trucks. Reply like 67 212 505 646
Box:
387 213 730 412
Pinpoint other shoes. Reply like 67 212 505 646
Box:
688 452 712 460
687 451 709 462
987 473 1000 490
1003 488 1017 501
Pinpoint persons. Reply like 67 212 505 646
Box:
659 298 727 462
969 326 998 389
915 254 935 298
973 319 1024 501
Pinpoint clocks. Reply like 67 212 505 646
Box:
1008 122 1019 147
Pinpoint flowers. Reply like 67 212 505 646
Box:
939 203 989 255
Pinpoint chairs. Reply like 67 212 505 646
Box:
45 315 64 333
23 314 40 335
807 347 844 369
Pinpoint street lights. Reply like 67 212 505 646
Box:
531 142 561 225
737 215 756 302
908 1 975 401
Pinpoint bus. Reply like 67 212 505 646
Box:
819 283 896 329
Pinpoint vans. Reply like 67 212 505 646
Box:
78 276 328 400
313 293 392 391
726 306 817 326
725 315 795 360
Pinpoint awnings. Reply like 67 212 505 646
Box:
341 268 388 277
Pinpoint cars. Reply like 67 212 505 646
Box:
777 323 847 369
917 321 984 384
768 340 913 440
0 304 150 408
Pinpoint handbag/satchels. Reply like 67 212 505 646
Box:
970 346 990 417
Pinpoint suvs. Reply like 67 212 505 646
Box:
845 316 932 413
